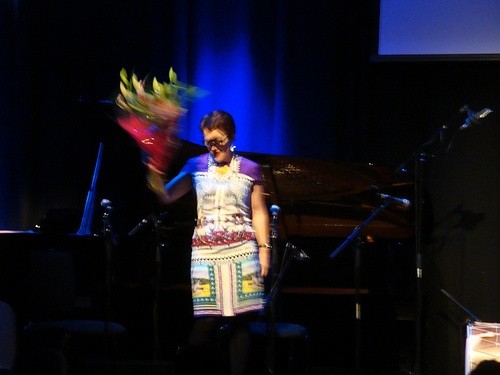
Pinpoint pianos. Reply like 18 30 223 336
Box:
93 100 420 374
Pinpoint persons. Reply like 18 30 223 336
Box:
467 358 499 375
145 108 273 375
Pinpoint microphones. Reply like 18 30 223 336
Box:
101 198 112 210
270 204 280 226
286 243 313 261
375 193 412 208
454 106 492 134
128 215 149 238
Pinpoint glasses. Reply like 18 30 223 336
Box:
203 135 229 148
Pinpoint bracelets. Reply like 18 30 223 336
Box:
257 243 273 250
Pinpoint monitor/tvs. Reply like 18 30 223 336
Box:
372 0 499 63
